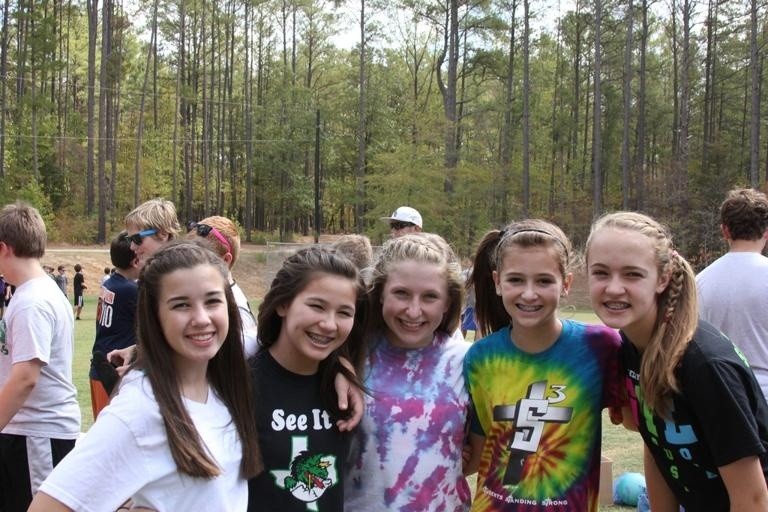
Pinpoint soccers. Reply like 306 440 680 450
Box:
617 473 646 505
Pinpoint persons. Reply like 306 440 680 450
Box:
332 235 373 271
184 216 263 362
0 204 81 511
380 206 424 239
27 240 364 511
694 188 768 401
0 264 116 355
89 230 145 425
460 252 483 343
246 246 370 510
584 212 768 511
106 195 182 377
339 231 475 511
464 217 642 512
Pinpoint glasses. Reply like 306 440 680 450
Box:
124 228 160 246
185 219 231 253
389 221 416 230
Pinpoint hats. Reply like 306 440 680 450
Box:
380 206 423 230
58 265 67 271
